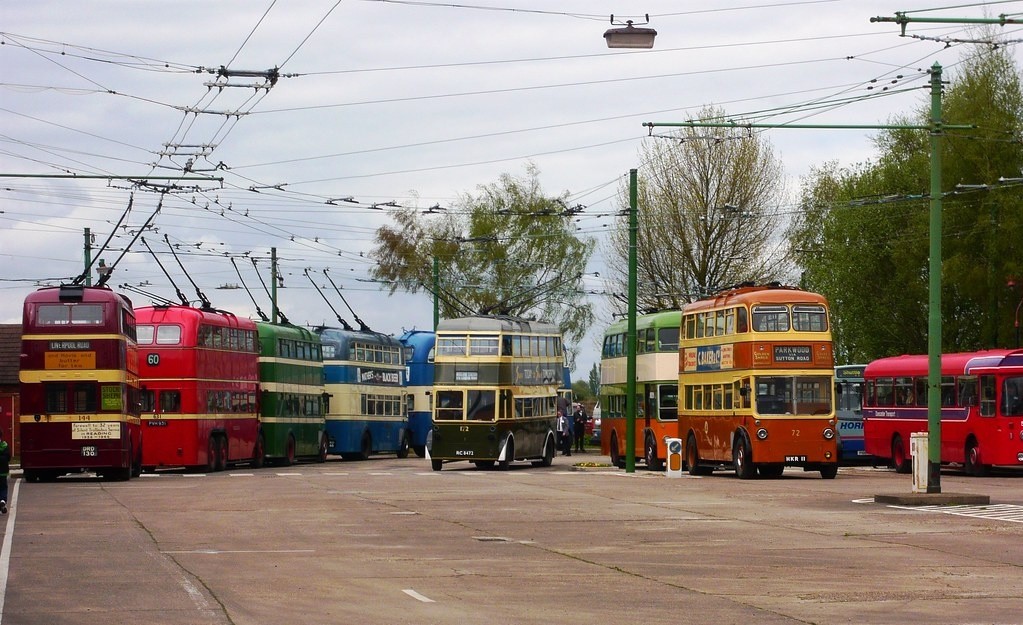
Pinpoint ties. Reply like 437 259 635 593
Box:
558 418 560 430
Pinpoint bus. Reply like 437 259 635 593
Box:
230 256 334 466
132 233 265 473
756 364 870 465
424 275 564 471
19 191 167 484
302 267 411 462
863 349 1023 478
599 293 683 471
676 281 845 481
397 281 575 453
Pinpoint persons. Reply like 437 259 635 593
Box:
556 409 571 456
573 404 587 453
0 429 11 514
867 381 916 407
584 416 595 435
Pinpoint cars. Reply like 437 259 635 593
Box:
592 400 601 440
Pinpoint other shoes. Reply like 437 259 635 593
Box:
574 450 577 452
580 450 585 452
566 454 571 456
